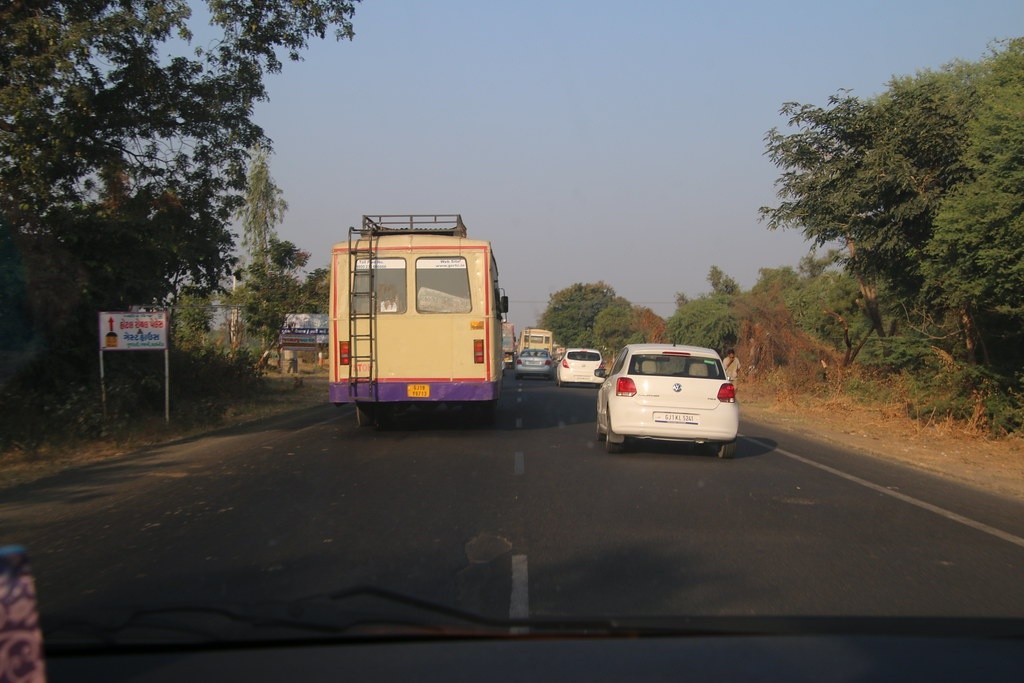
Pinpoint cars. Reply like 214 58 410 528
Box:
554 348 607 388
514 348 556 380
594 343 740 460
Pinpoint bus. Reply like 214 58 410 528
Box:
501 323 516 371
516 327 553 359
328 214 509 423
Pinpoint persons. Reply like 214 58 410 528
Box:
722 349 741 396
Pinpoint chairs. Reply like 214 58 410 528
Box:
641 360 657 375
688 363 709 377
